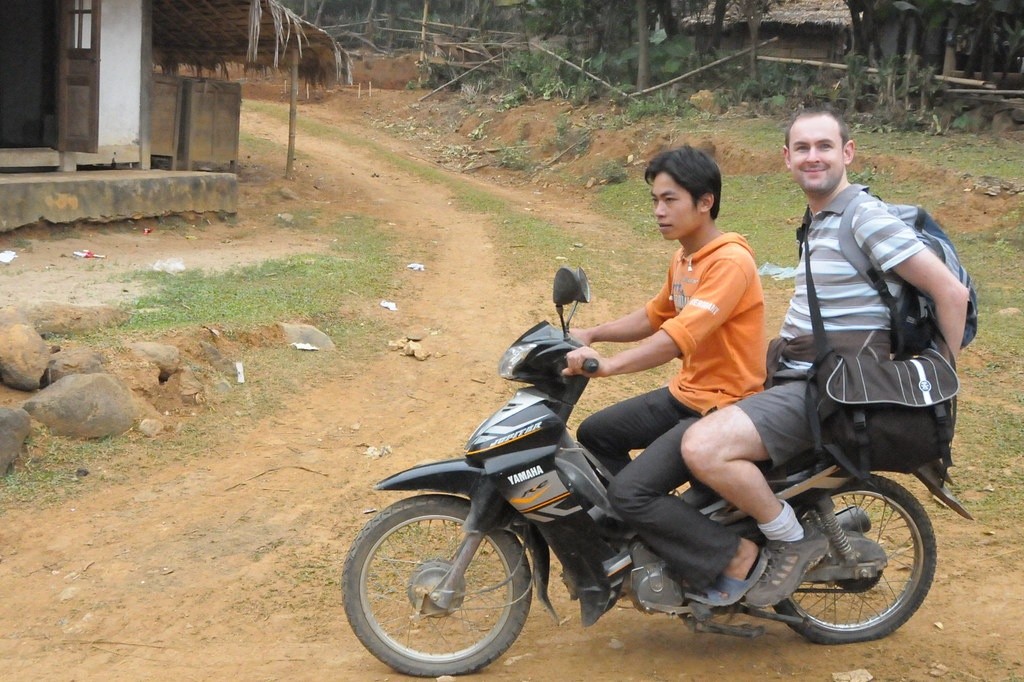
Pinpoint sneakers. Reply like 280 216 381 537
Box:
742 519 831 610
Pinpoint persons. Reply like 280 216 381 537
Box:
562 146 770 607
680 108 969 607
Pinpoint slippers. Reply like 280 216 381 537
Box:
685 549 768 607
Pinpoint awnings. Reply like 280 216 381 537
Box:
152 0 352 181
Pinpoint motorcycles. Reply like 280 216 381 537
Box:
341 266 975 678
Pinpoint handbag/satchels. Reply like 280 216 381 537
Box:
807 345 962 484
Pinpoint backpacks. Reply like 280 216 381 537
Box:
842 191 979 360
